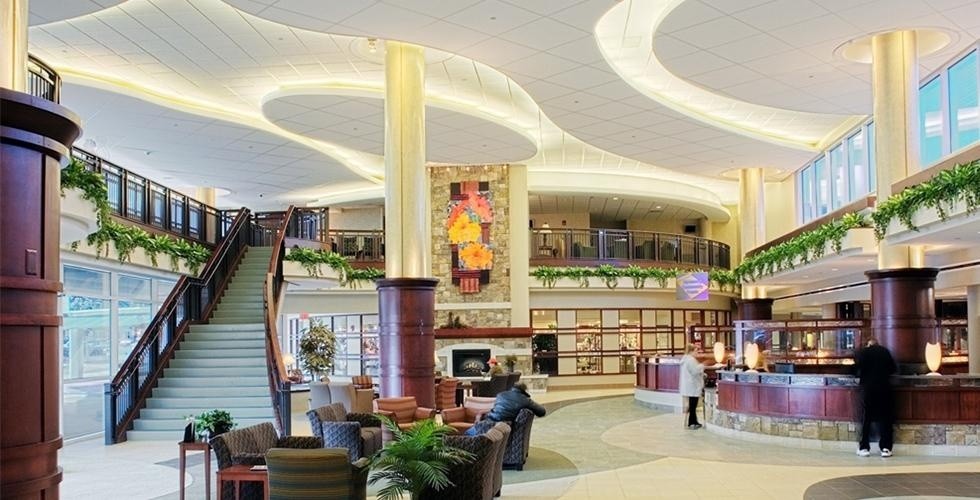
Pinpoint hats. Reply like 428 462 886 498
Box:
486 357 497 364
512 381 531 398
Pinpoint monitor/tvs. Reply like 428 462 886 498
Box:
775 363 794 374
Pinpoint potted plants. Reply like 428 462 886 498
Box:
183 409 239 443
297 317 341 381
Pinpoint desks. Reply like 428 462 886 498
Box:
177 440 213 500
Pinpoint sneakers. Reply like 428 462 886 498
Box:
854 447 871 457
879 449 894 457
688 422 703 428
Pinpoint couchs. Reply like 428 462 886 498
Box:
210 373 534 499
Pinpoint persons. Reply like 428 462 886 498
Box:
853 336 897 457
752 342 770 374
679 343 708 429
463 379 546 435
